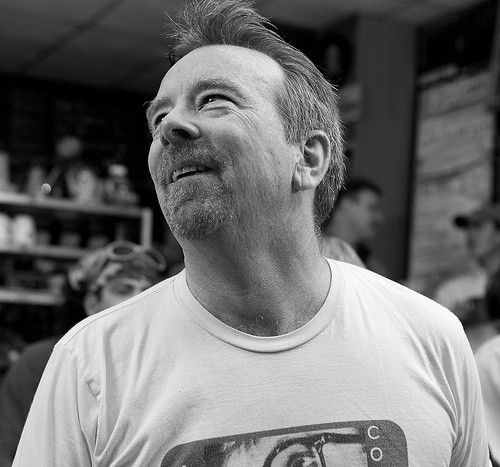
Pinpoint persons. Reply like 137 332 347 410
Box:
0 239 165 467
322 178 500 467
10 0 491 467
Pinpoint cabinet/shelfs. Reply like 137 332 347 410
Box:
312 14 417 282
0 193 152 306
